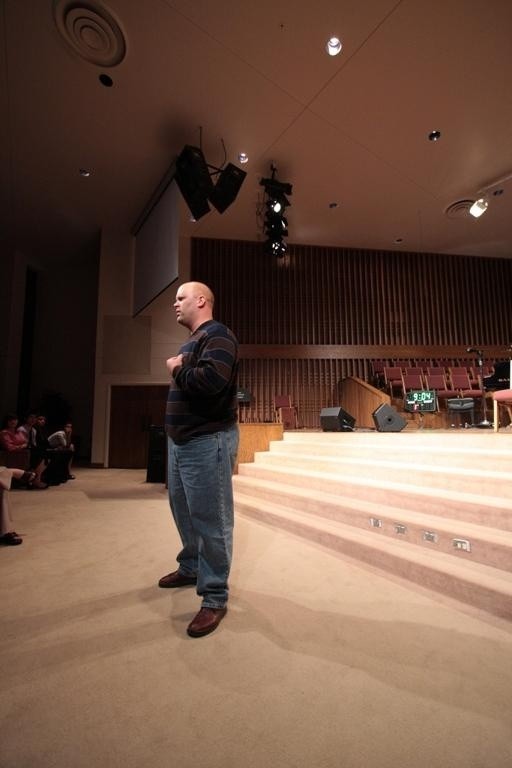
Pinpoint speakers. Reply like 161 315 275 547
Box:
320 407 356 431
209 163 249 216
177 151 211 222
372 402 409 432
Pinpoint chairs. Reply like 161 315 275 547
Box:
272 395 298 427
370 359 488 412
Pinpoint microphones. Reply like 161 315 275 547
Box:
466 347 485 353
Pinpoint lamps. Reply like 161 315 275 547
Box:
259 165 294 258
468 192 490 217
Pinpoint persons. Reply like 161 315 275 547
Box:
0 410 51 489
157 278 240 640
18 411 38 451
48 420 77 479
0 465 38 546
30 412 50 447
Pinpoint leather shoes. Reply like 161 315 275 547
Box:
0 533 22 544
186 606 228 637
21 471 36 480
159 571 198 587
